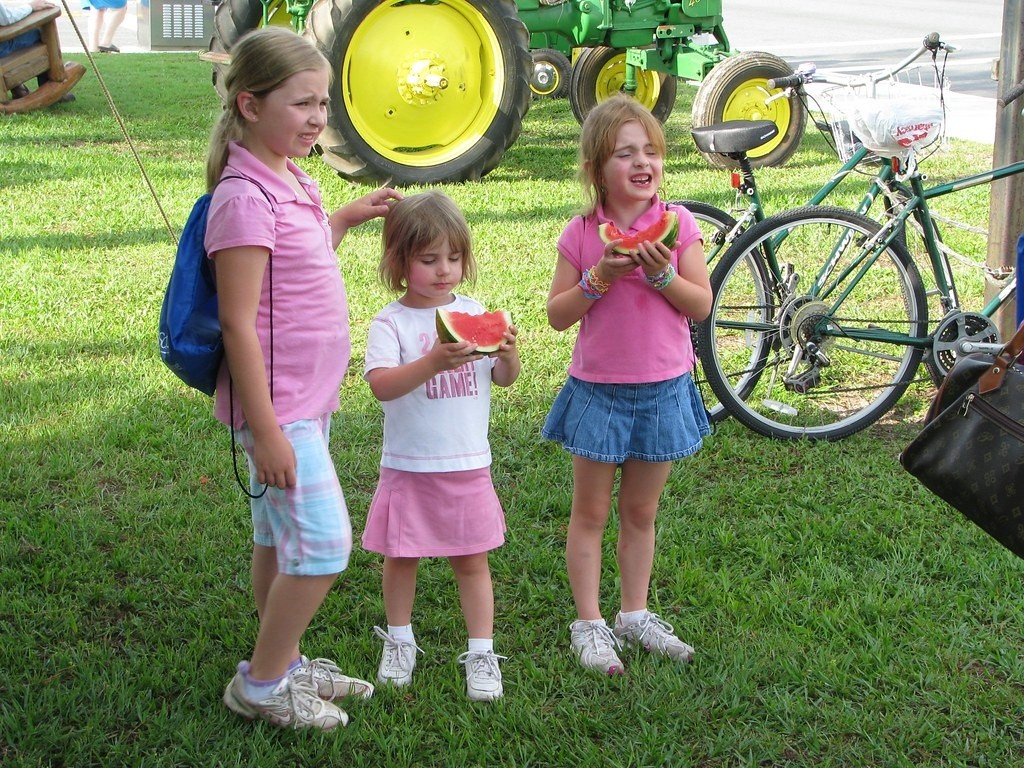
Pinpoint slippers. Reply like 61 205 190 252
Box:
97 44 120 52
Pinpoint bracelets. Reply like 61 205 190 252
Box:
577 264 611 300
645 262 675 290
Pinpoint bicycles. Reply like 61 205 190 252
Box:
694 79 1024 441
667 32 961 426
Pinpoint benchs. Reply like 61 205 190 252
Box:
0 6 86 113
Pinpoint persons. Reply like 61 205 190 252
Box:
203 29 520 729
90 0 127 52
0 0 76 104
545 101 712 671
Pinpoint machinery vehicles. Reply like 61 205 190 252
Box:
196 1 808 190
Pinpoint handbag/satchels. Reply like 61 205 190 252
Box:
832 88 942 182
899 318 1024 560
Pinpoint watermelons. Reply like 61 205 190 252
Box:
598 211 679 258
435 308 514 356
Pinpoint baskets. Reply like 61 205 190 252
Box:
819 61 953 168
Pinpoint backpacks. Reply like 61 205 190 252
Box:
155 173 275 398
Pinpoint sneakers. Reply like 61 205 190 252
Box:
569 620 623 678
372 624 425 687
457 648 508 703
289 655 375 703
224 661 348 734
611 610 696 664
